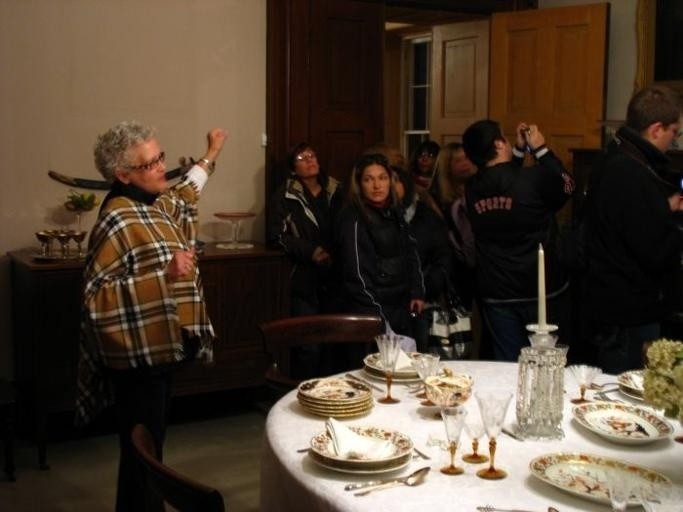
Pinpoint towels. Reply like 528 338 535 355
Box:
322 415 392 464
376 345 412 372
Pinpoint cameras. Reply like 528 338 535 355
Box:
520 127 531 139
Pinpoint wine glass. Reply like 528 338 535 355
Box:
374 333 404 406
33 229 87 258
440 390 513 480
423 369 474 417
566 364 602 408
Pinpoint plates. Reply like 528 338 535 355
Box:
572 400 675 445
529 453 673 507
296 350 439 476
615 367 649 401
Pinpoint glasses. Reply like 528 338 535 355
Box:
129 151 166 172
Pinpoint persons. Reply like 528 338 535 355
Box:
331 141 480 367
264 141 344 314
580 85 682 375
70 118 230 511
462 119 579 363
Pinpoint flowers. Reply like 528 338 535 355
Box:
638 336 683 430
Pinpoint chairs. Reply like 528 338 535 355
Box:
116 407 229 511
259 312 382 393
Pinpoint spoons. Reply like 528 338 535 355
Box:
343 468 429 497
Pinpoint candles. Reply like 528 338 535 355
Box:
535 239 551 324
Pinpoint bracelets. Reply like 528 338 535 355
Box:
200 157 215 173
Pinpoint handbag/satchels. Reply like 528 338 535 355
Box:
423 299 482 360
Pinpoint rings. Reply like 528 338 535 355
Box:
184 264 189 270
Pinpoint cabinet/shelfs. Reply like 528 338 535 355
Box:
3 238 290 432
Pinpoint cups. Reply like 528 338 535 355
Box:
605 479 683 511
516 345 567 444
